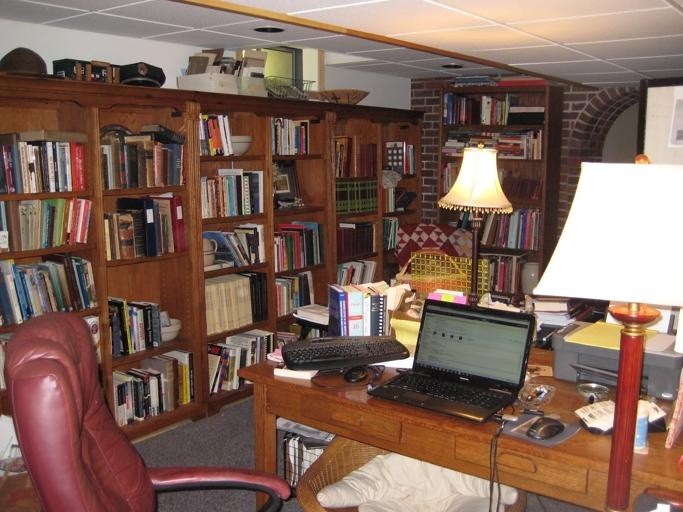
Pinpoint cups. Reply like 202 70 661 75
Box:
633 399 649 449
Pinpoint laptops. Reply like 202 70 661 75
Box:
367 298 535 422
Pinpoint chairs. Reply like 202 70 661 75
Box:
1 309 292 512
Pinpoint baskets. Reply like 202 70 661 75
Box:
264 76 315 100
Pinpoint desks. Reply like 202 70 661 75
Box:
235 343 681 512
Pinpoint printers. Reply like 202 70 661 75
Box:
552 306 683 400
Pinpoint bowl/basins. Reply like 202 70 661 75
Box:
161 319 181 342
230 135 254 157
320 89 370 105
517 383 556 411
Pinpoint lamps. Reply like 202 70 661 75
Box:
532 156 682 511
437 146 514 308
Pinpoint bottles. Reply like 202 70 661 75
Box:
521 263 539 295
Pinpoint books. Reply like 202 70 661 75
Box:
199 113 274 394
527 293 599 328
266 281 421 379
448 75 546 87
270 117 325 349
101 124 194 427
334 135 414 284
443 93 541 308
0 133 104 477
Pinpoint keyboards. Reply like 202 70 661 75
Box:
282 335 409 370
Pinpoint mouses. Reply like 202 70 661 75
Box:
527 417 565 440
345 366 368 383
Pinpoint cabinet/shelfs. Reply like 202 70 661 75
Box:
432 81 559 308
